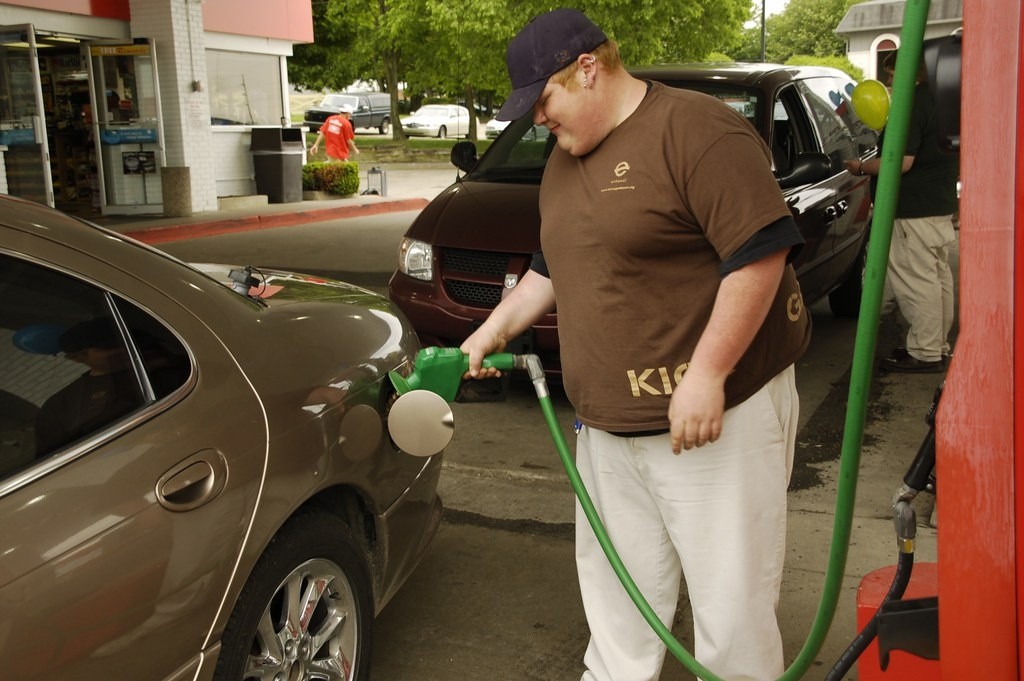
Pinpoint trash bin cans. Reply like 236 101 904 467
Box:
248 126 306 204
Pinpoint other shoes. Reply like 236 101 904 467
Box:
892 345 949 366
880 357 944 373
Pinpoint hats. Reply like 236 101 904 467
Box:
339 104 354 115
495 8 607 122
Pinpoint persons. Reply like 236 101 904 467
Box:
37 313 190 457
843 46 958 372
311 104 359 164
461 10 812 681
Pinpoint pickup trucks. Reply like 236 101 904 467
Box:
301 94 391 135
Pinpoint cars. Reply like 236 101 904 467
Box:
400 103 483 138
486 114 549 141
390 64 883 382
0 196 446 681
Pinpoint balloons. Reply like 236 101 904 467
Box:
851 79 890 131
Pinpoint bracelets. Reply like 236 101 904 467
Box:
859 162 863 175
